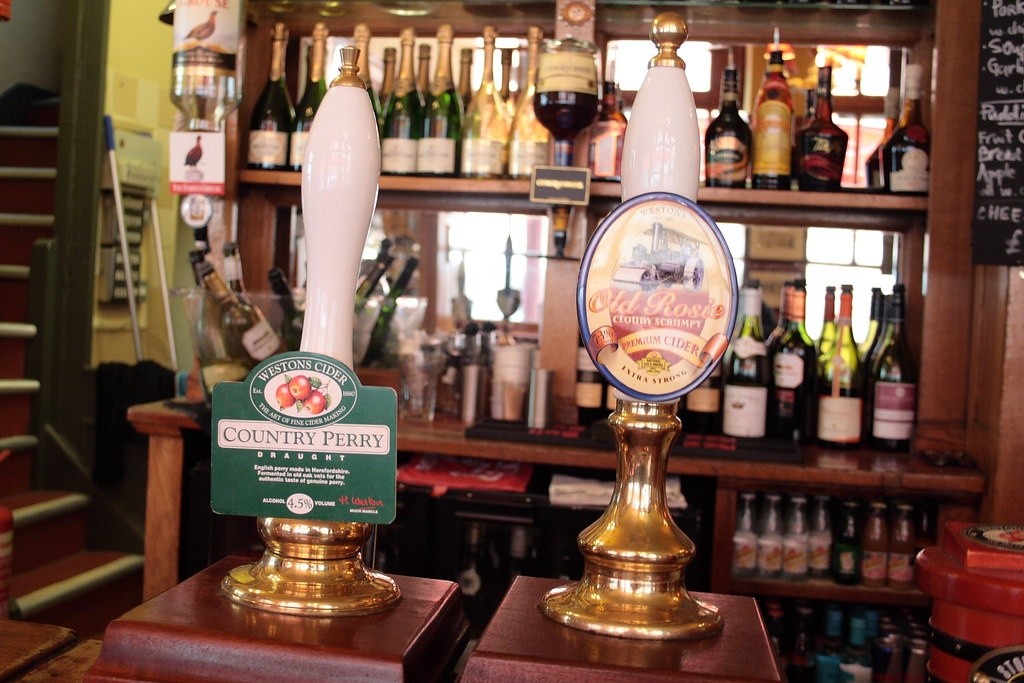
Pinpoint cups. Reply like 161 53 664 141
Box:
491 343 528 419
453 357 491 429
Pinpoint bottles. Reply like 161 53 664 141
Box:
731 494 917 590
533 37 599 261
588 82 628 183
573 280 914 450
764 602 928 683
862 86 908 194
453 520 490 636
170 1 242 256
501 526 529 586
883 64 930 197
751 50 795 190
796 67 848 192
705 69 750 188
248 21 550 180
190 237 419 368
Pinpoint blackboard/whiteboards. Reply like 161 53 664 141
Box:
971 0 1024 265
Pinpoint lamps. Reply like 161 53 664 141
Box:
534 12 726 637
223 47 404 616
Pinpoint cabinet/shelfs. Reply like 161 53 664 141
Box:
125 0 992 608
